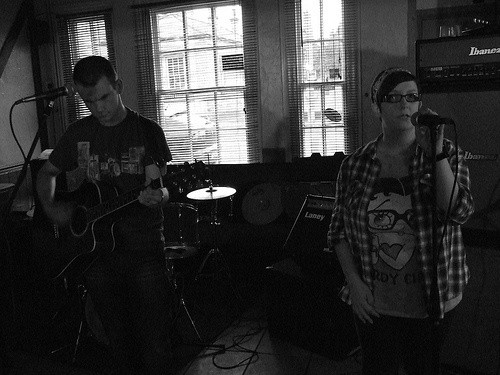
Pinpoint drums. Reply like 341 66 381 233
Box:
238 179 289 230
82 286 129 350
161 202 201 262
186 185 239 221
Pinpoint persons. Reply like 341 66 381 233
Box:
37 56 179 374
326 67 477 375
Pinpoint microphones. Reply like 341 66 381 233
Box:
15 84 74 105
411 112 454 126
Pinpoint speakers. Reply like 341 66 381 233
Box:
418 80 500 231
282 194 342 275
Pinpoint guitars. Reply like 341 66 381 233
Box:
33 160 215 289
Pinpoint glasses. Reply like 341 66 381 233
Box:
383 93 423 103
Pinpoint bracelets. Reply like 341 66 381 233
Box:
158 188 164 205
426 152 449 165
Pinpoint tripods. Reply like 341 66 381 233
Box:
186 185 246 308
